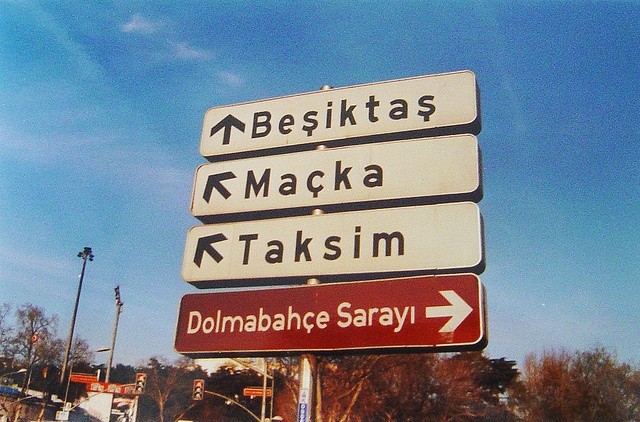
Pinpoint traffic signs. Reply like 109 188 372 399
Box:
171 272 490 359
199 70 482 163
243 388 272 398
181 200 487 290
188 131 484 225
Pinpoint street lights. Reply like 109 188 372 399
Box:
62 348 111 412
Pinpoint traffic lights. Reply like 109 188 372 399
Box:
133 373 147 395
192 379 205 401
